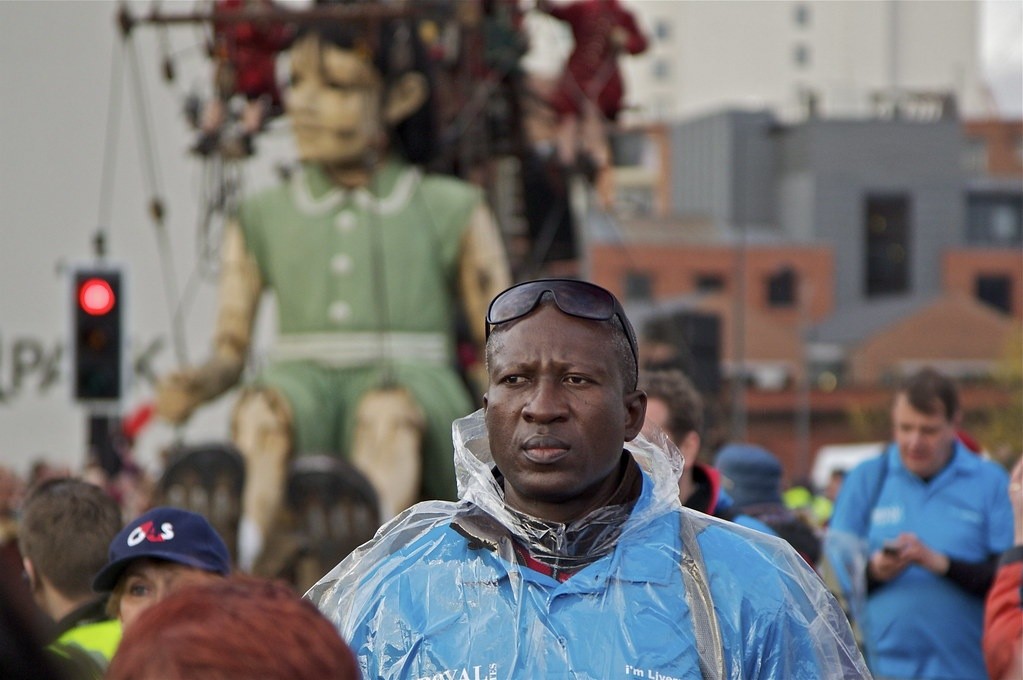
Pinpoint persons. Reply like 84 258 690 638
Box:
983 463 1023 680
15 476 125 680
823 366 1015 680
718 443 845 572
106 575 358 680
194 0 289 160
92 506 229 628
303 278 873 680
154 15 513 570
638 367 776 536
537 0 649 157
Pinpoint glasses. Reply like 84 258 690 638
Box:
485 278 639 391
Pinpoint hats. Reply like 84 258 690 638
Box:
713 444 783 505
92 506 228 594
